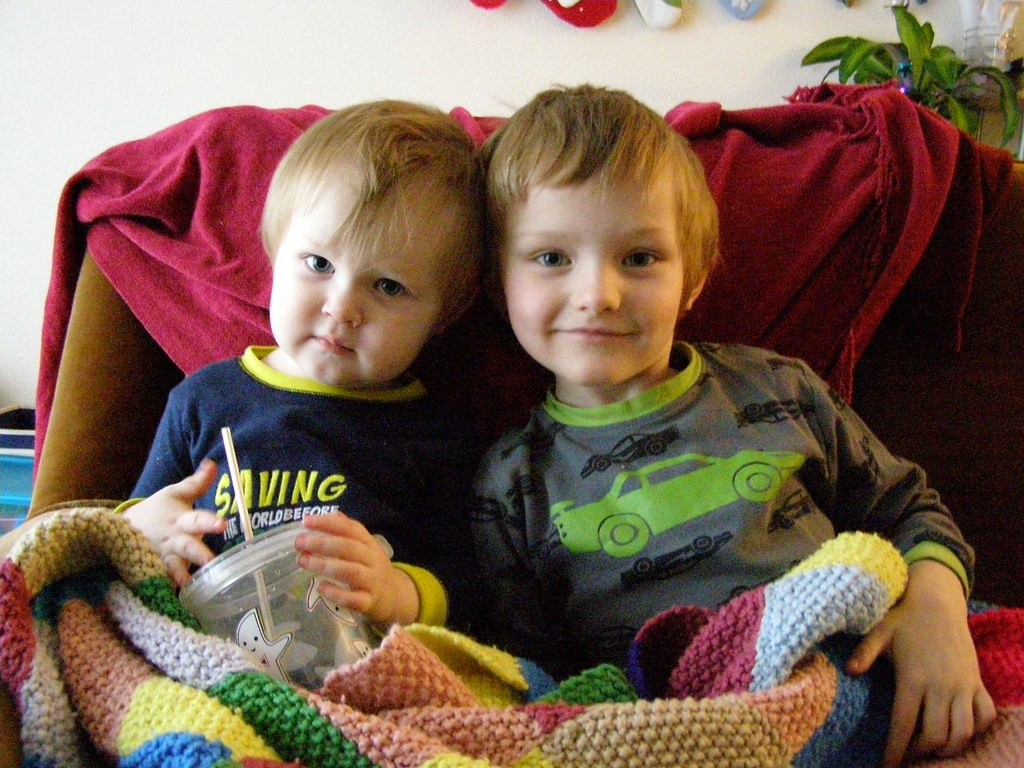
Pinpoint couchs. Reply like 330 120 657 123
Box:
36 103 1023 598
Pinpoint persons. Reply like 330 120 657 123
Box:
111 101 497 691
469 83 996 768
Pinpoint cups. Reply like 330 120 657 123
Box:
179 520 378 691
